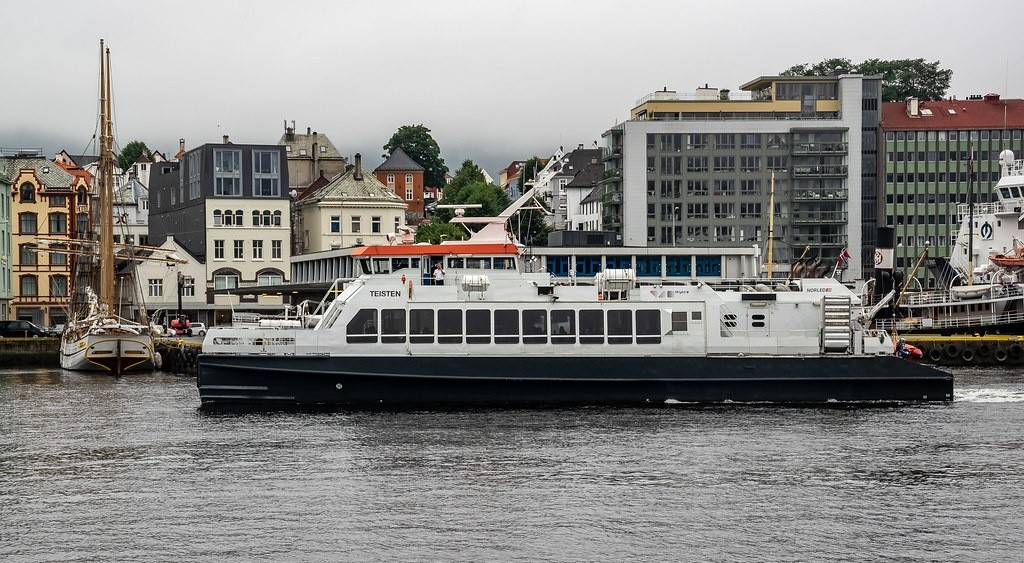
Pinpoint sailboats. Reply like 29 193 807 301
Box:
59 38 160 373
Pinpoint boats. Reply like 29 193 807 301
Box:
195 243 960 407
951 282 1003 298
871 148 1024 367
988 247 1024 267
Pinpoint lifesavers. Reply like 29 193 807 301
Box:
915 343 927 354
164 348 201 370
928 348 942 362
1008 344 1022 358
993 348 1007 361
961 348 974 362
945 344 960 357
409 279 413 299
977 343 991 357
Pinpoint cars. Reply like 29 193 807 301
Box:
0 320 51 337
50 323 64 338
36 324 50 333
190 322 207 337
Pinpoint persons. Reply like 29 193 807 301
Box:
433 263 445 285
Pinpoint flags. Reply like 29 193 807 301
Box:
837 247 851 267
